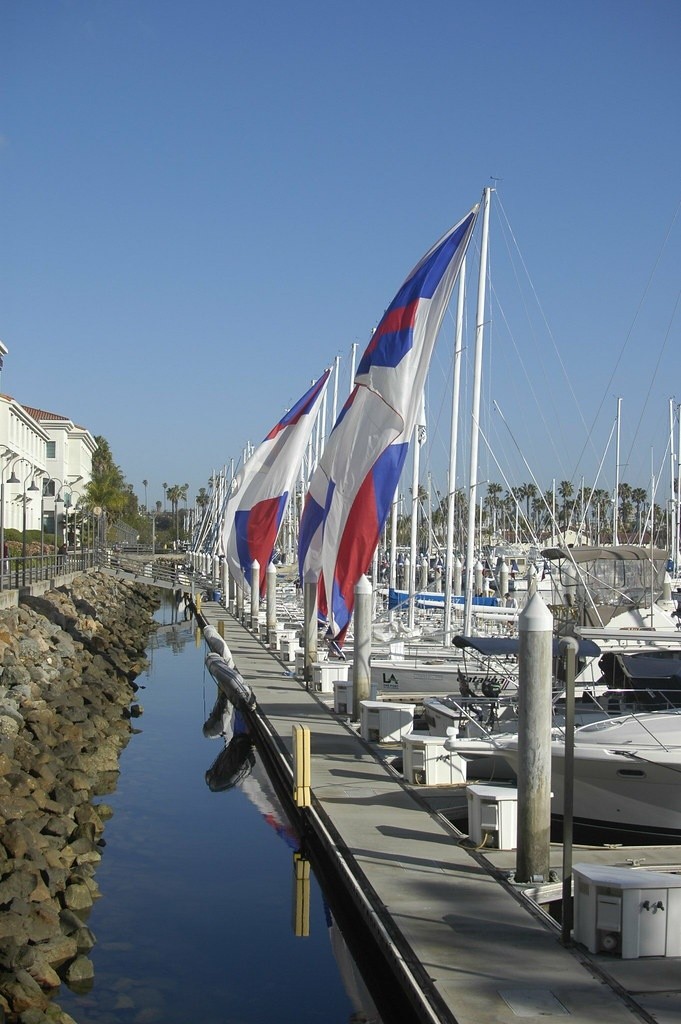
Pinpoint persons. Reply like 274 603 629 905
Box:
56 543 69 574
0 539 9 574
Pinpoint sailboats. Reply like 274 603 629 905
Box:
189 174 681 849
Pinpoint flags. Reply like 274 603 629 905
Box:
221 211 481 654
222 699 303 853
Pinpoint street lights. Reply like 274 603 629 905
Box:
27 469 50 561
6 458 33 562
53 485 99 560
